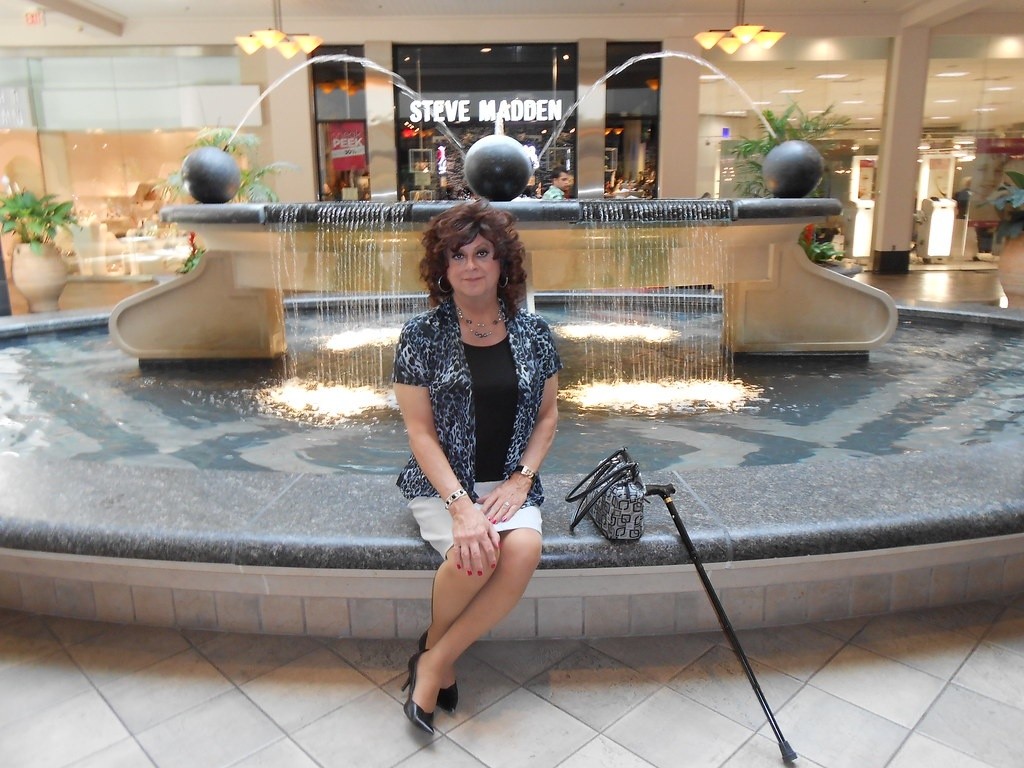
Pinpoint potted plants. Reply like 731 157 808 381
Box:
972 170 1024 295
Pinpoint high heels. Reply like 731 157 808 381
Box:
417 630 459 712
401 649 436 736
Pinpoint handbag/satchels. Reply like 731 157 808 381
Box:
566 446 646 541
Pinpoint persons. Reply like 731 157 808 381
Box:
973 153 1008 220
536 180 553 198
952 177 971 219
928 158 950 199
859 165 875 200
391 195 563 735
541 167 569 199
605 162 657 199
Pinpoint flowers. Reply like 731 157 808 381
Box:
0 173 80 246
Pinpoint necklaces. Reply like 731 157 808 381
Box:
454 303 503 338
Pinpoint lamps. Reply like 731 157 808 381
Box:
694 0 785 56
236 0 321 59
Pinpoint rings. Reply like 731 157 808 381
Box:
505 502 509 506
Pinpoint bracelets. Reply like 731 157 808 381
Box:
445 489 467 510
515 465 535 479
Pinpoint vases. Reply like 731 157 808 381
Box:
10 241 68 314
